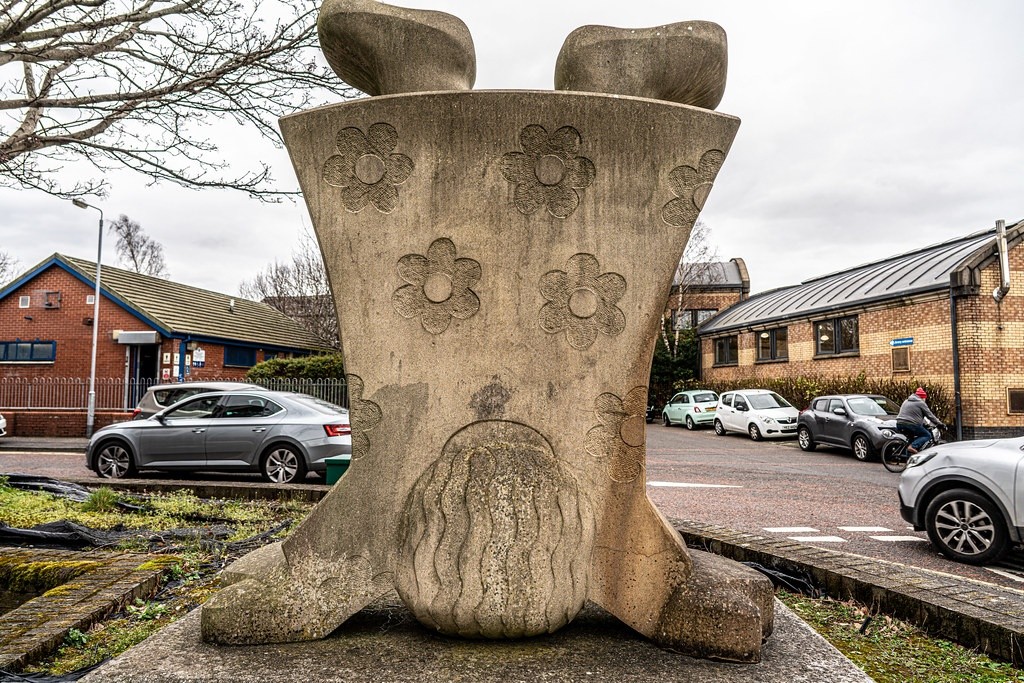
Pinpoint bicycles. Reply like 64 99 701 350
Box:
881 424 950 473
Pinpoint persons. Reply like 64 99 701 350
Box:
896 387 946 454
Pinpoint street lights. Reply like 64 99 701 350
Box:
73 198 104 439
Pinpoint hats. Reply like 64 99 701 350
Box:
916 388 927 398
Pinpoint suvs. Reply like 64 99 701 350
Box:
130 382 268 420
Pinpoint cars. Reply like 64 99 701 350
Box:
661 390 719 430
897 435 1024 563
713 389 801 441
85 391 351 484
797 394 940 461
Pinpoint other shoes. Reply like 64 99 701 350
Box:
908 444 918 453
